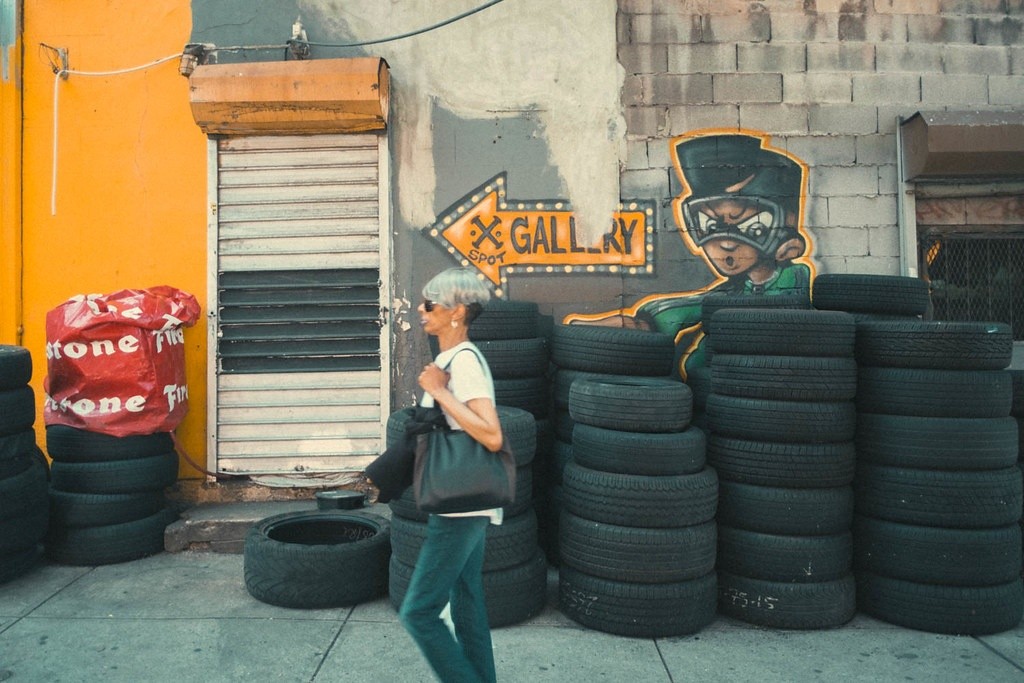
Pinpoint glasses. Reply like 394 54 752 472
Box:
424 299 453 313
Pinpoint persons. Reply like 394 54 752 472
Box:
364 266 503 683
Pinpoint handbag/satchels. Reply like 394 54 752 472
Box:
412 346 515 512
